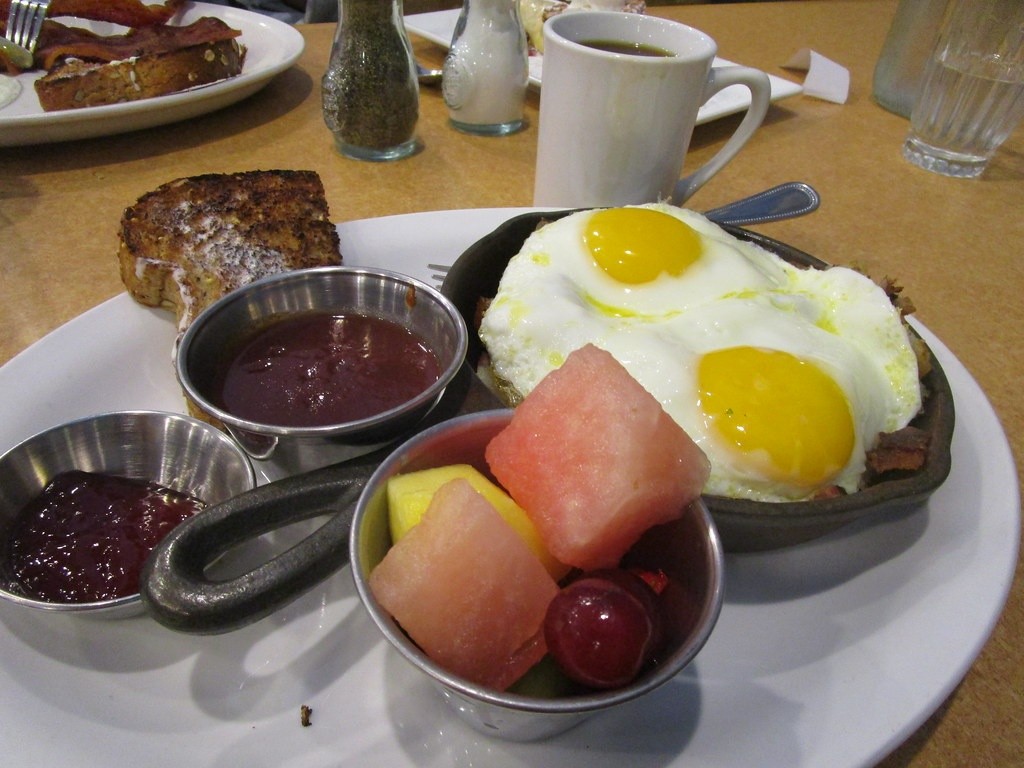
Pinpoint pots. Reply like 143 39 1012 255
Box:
142 204 956 635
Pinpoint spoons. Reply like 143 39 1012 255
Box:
415 65 442 85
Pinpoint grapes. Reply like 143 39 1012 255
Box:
542 579 654 689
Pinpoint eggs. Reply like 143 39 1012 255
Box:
476 199 924 502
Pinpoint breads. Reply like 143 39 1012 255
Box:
117 170 345 428
34 15 244 112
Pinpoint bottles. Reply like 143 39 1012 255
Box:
441 0 528 135
321 0 420 162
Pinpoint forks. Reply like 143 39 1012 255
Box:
7 0 50 56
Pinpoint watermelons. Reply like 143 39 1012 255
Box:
484 344 712 571
366 479 560 694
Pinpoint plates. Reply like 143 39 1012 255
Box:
0 206 1024 768
403 5 802 127
0 1 305 148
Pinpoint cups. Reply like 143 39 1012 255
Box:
870 0 949 122
349 409 728 745
0 409 256 615
903 0 1024 178
175 265 468 483
533 11 772 211
